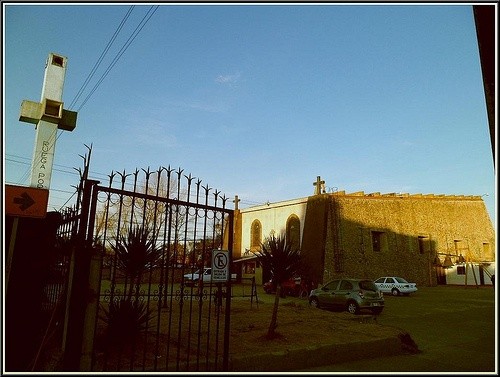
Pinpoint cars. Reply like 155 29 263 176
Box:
182 267 224 287
374 276 419 297
309 277 384 316
263 275 309 297
